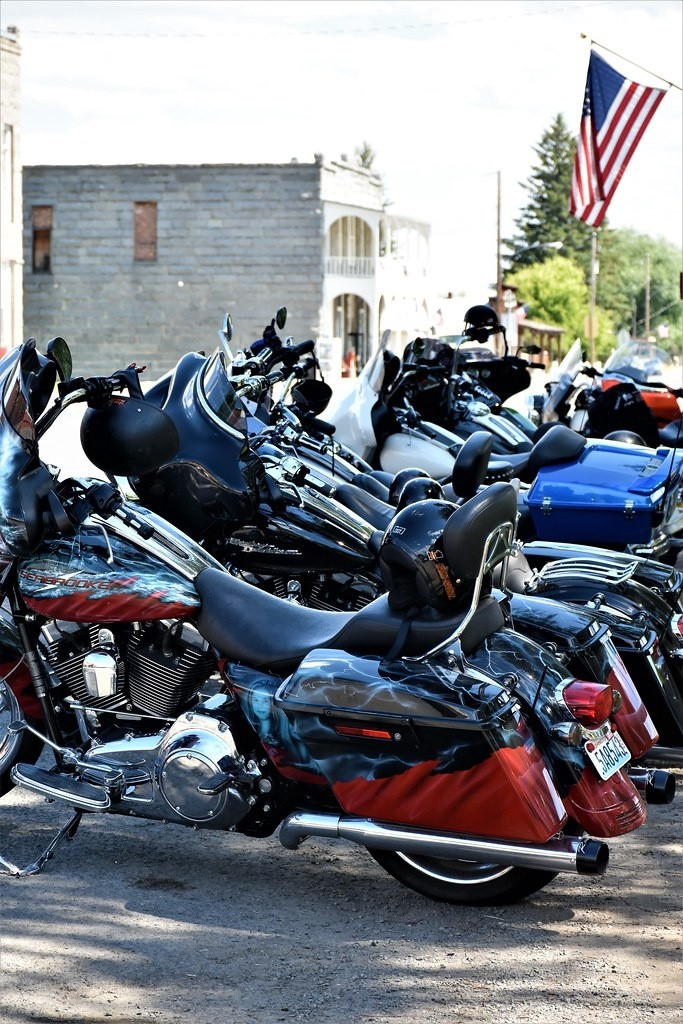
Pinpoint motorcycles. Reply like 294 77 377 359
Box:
0 336 648 908
117 302 683 813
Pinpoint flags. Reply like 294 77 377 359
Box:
568 49 666 229
